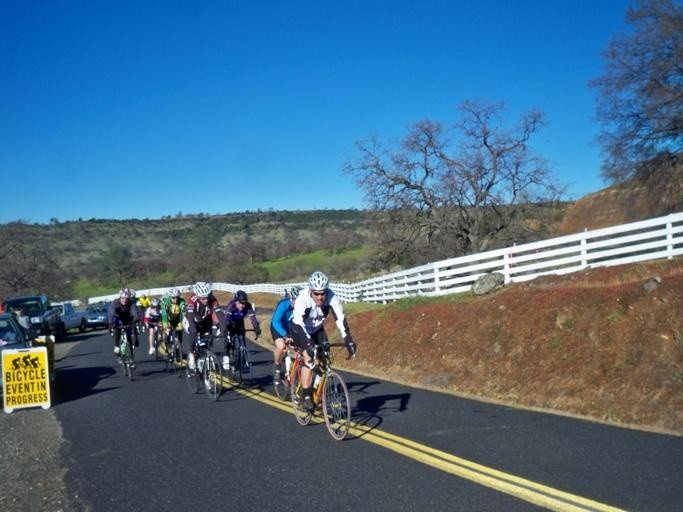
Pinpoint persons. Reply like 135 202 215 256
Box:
271 285 304 383
108 281 261 379
291 271 356 410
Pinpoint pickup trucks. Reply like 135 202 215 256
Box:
2 296 60 336
50 302 87 341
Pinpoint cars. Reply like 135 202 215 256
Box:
0 311 39 399
83 301 112 328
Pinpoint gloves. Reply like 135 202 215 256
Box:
344 336 356 354
304 339 314 358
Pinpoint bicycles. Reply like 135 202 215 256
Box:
115 325 135 381
148 322 256 401
273 340 350 440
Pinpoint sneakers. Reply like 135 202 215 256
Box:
222 356 229 370
187 352 197 370
273 364 282 386
300 387 314 410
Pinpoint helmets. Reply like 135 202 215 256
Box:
119 288 131 298
309 271 329 290
235 291 246 304
168 288 181 297
285 287 299 299
129 289 136 298
194 282 212 298
151 297 160 307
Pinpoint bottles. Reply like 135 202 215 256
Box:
310 371 322 390
284 355 293 374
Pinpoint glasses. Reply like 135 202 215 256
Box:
312 291 326 296
